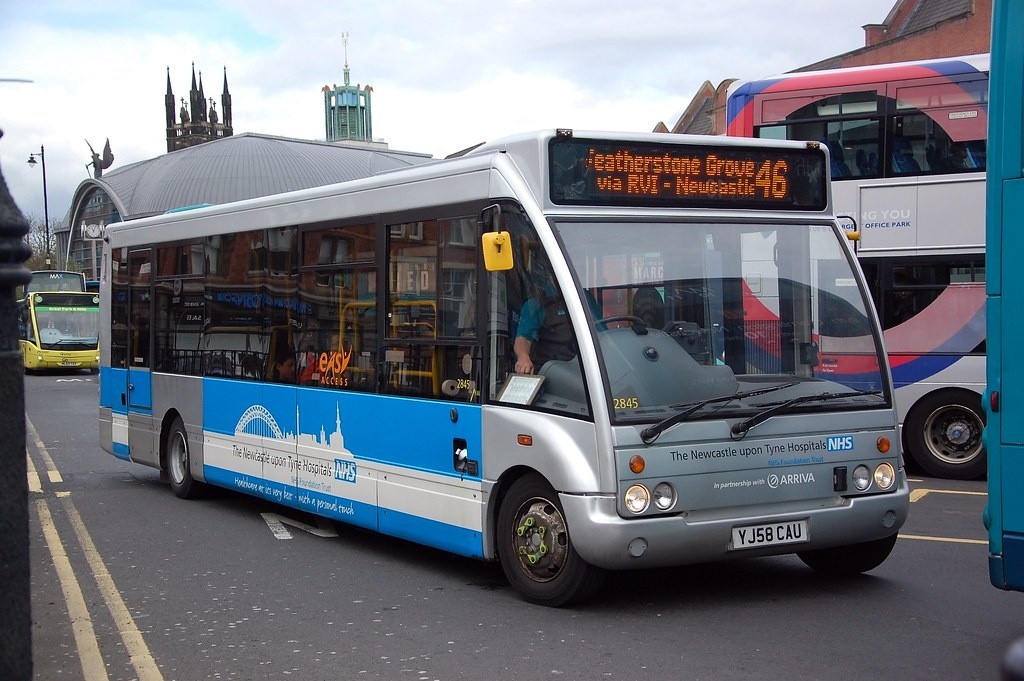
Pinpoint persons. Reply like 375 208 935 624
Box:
87 153 102 179
514 265 609 375
937 138 967 172
300 333 351 387
273 349 295 382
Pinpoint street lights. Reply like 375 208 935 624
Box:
27 152 51 269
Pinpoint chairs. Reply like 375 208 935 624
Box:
926 145 941 171
892 133 913 173
968 140 986 167
867 152 878 175
855 149 872 175
826 141 852 177
903 138 922 172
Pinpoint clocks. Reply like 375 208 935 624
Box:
86 224 100 238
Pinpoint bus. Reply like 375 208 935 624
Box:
723 53 989 477
97 128 911 606
989 0 1024 594
86 281 101 292
22 273 86 295
17 291 98 374
